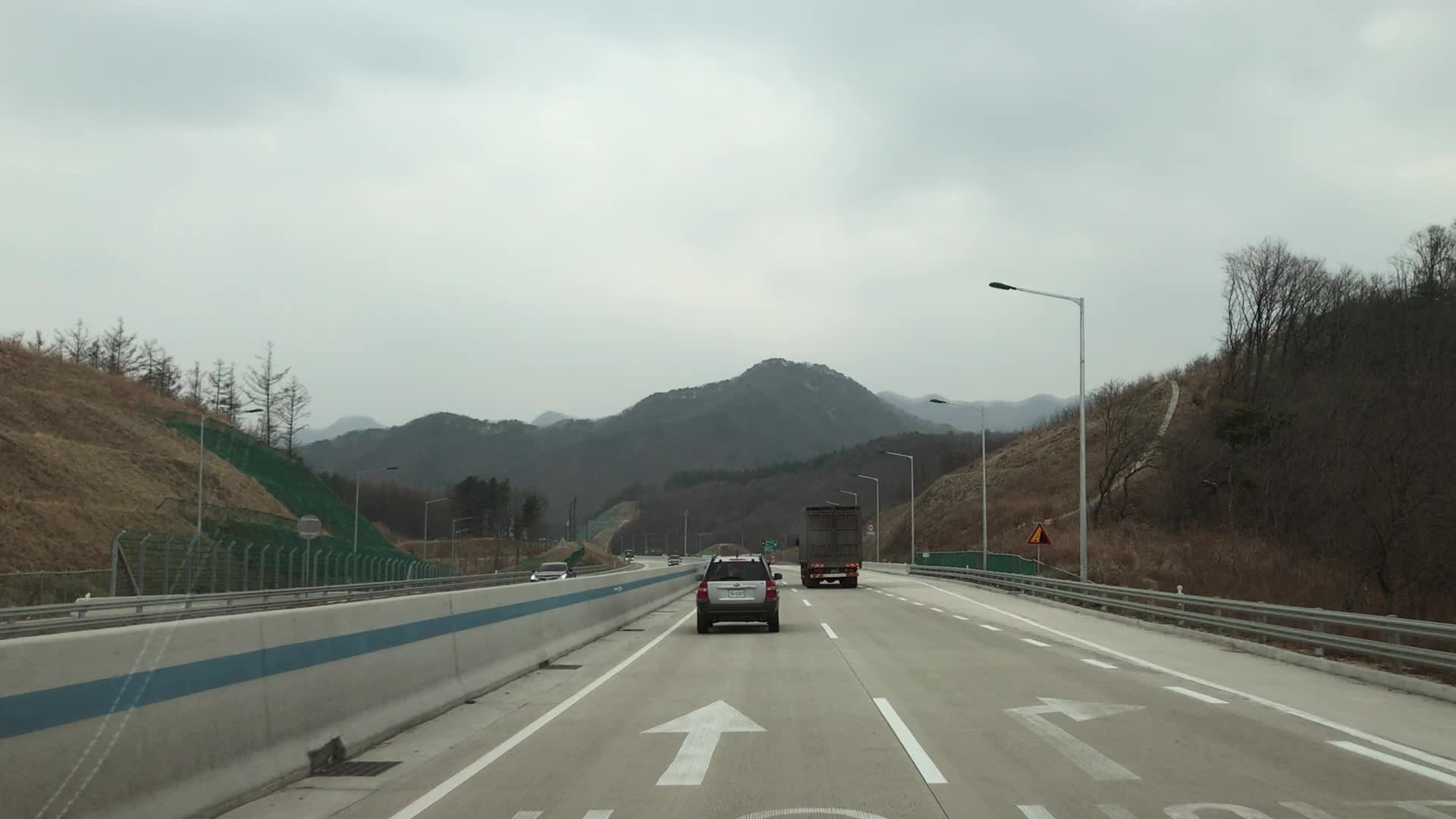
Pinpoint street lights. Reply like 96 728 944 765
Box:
455 528 471 564
873 449 915 564
838 489 858 504
927 397 988 574
193 408 265 563
683 509 690 557
451 516 473 560
850 472 880 562
351 465 401 583
423 497 450 563
989 280 1089 598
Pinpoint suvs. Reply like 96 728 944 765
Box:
695 551 784 633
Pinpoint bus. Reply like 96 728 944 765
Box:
625 550 634 561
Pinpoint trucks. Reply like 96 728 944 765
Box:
795 505 863 589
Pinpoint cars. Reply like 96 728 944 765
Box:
668 554 682 566
529 561 577 583
742 553 773 572
